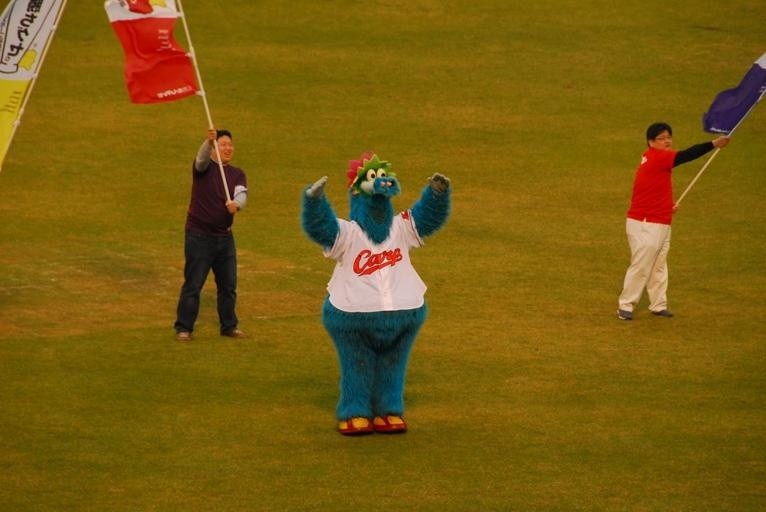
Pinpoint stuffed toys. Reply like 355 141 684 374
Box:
299 149 455 435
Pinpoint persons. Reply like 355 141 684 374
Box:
613 123 732 320
172 124 248 342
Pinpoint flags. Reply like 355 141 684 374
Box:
101 0 198 104
702 51 766 137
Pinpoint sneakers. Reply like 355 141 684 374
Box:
177 331 192 341
220 328 244 337
616 308 632 320
654 309 671 316
